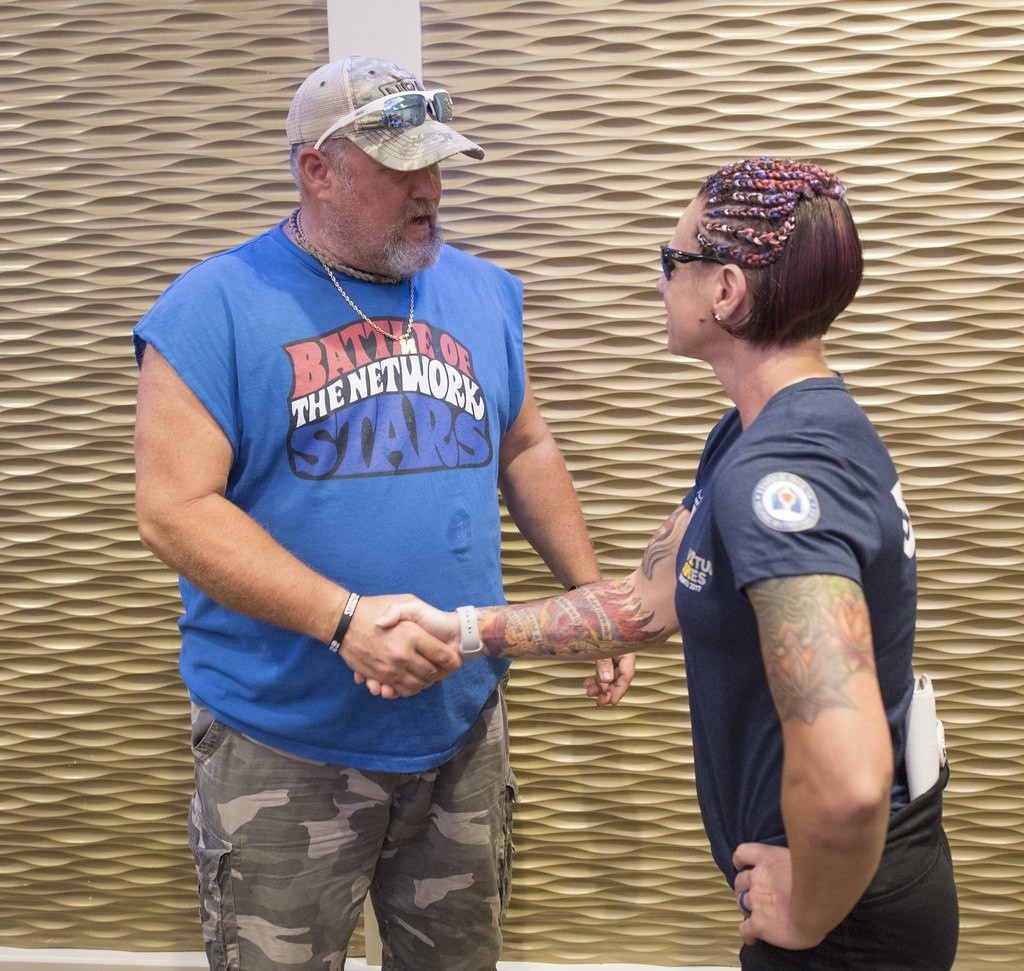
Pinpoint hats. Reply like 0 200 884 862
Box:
286 57 485 173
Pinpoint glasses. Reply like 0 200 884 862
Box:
661 246 743 281
313 88 453 152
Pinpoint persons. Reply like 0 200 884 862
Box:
351 156 961 971
133 57 637 970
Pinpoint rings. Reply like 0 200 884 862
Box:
739 891 753 913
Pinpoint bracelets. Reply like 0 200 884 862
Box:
455 605 484 658
329 592 362 655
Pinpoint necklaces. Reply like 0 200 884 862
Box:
297 206 414 341
